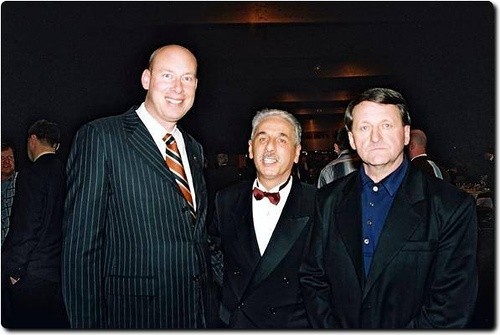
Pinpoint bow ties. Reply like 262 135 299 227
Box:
252 175 291 205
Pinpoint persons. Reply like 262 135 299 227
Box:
213 107 319 332
298 88 479 331
1 119 67 330
1 139 19 332
195 129 497 224
59 45 217 331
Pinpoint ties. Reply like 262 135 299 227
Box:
162 133 194 209
